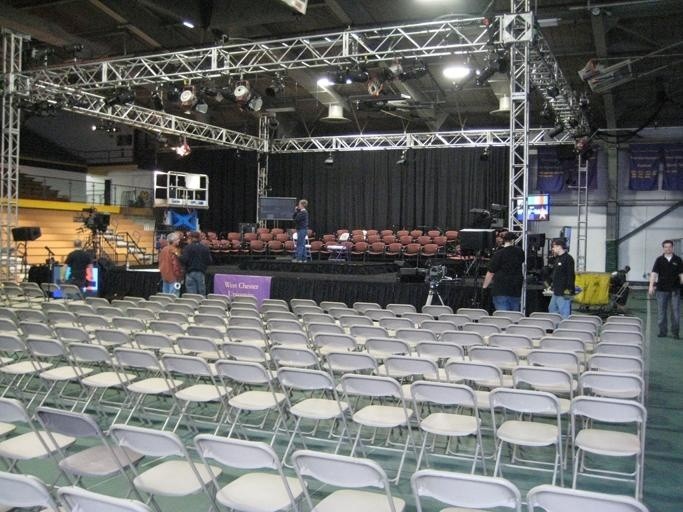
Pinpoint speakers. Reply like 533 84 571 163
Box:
398 267 425 282
11 226 42 241
459 228 497 252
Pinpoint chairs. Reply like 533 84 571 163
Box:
158 227 460 269
0 281 649 512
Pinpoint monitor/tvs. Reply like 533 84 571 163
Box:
517 194 551 223
52 264 100 297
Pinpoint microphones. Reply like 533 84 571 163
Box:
45 246 55 256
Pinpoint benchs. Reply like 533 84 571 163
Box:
0 166 71 205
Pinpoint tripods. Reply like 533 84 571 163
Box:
81 231 113 261
425 289 445 307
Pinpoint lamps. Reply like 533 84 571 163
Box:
317 58 426 88
323 151 335 164
542 73 603 168
444 51 510 91
396 150 407 166
156 134 191 156
89 117 120 137
10 72 288 115
481 148 490 161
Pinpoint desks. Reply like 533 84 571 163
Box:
460 228 497 268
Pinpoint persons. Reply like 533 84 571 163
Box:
65 240 92 292
547 239 575 319
168 231 213 296
648 240 683 339
482 232 526 312
292 199 308 262
159 233 183 297
608 265 632 314
